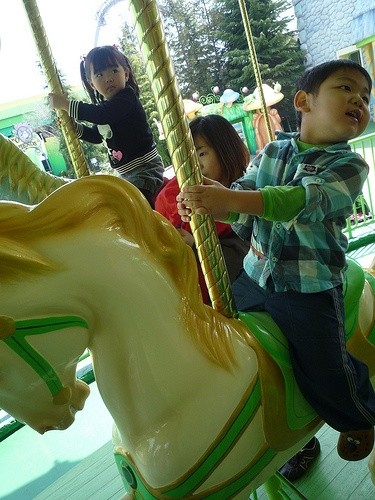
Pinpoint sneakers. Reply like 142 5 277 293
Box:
280 435 320 480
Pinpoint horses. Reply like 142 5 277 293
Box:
0 134 375 500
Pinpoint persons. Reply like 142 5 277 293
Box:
155 114 321 483
175 58 375 462
48 43 165 210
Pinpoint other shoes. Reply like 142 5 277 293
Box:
337 426 374 461
